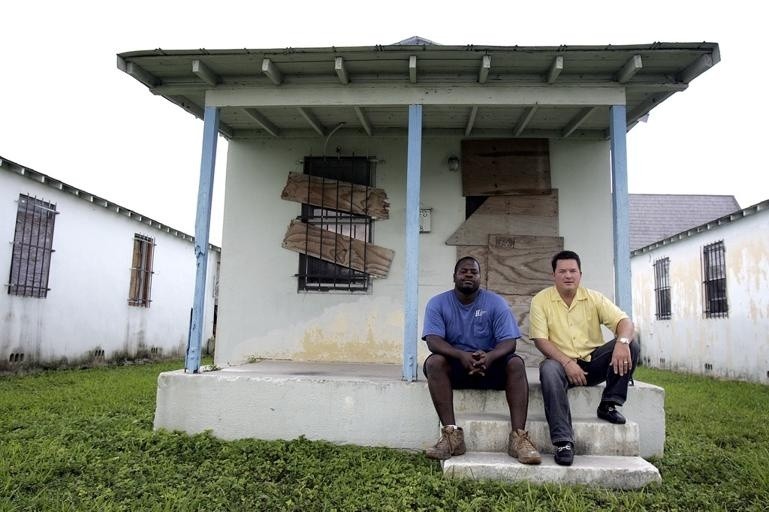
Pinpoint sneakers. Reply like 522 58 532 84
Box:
597 406 626 424
424 425 466 460
554 444 574 466
507 428 542 464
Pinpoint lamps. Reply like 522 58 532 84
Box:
447 154 460 171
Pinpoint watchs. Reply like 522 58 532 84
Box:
616 336 632 344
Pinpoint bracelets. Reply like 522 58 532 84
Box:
562 359 572 367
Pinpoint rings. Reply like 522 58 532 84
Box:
624 362 628 364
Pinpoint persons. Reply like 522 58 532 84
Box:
528 251 634 465
420 255 542 465
